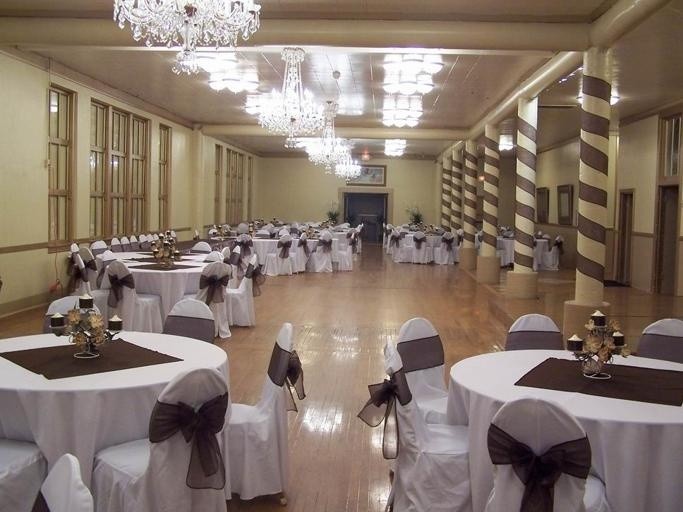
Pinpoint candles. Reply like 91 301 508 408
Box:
567 310 624 351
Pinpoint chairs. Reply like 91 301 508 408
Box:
384 334 470 512
218 322 293 506
383 223 564 271
637 318 683 365
484 399 612 511
0 439 94 511
396 317 449 424
91 365 230 512
505 314 563 350
